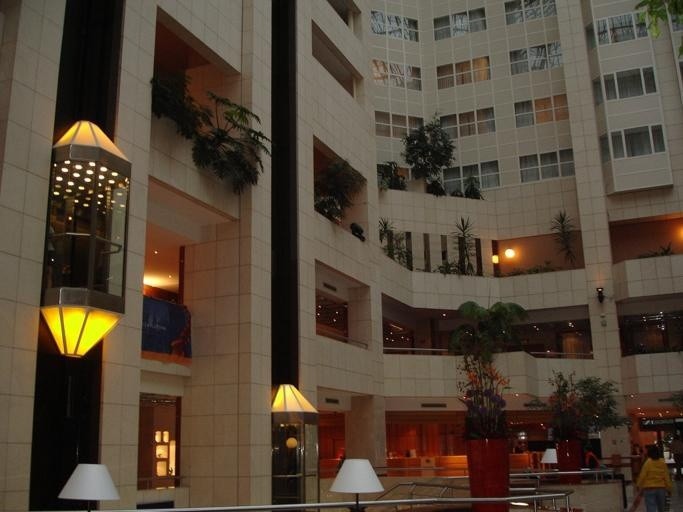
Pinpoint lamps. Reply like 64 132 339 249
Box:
57 463 121 511
326 457 384 512
540 449 559 472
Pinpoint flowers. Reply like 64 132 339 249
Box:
534 362 638 439
453 347 514 440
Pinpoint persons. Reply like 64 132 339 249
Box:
45 222 57 289
515 441 523 453
585 444 600 481
631 444 674 511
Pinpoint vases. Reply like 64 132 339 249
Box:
554 439 585 486
464 441 513 512
